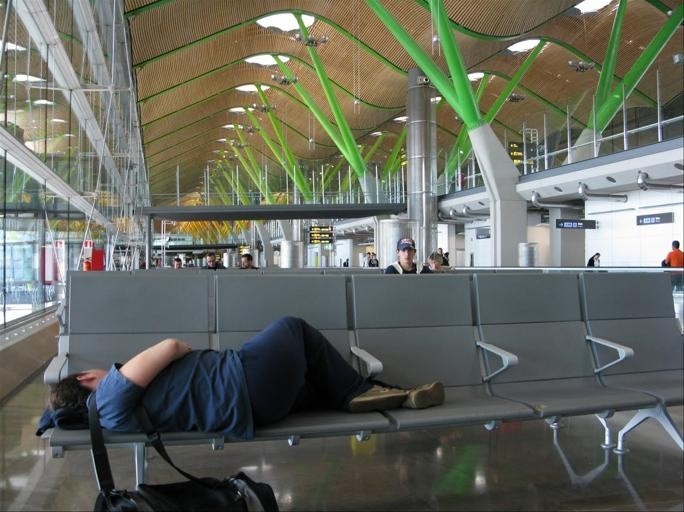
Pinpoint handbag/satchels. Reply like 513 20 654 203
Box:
94 471 279 512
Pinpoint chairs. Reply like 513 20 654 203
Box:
52 269 681 512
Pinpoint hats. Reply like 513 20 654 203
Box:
397 237 416 250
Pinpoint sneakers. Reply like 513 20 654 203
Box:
349 381 447 413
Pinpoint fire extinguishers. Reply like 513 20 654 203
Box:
83 257 96 271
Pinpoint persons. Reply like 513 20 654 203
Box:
173 252 257 271
344 237 452 273
662 260 669 268
587 253 601 267
50 316 444 439
665 240 684 292
